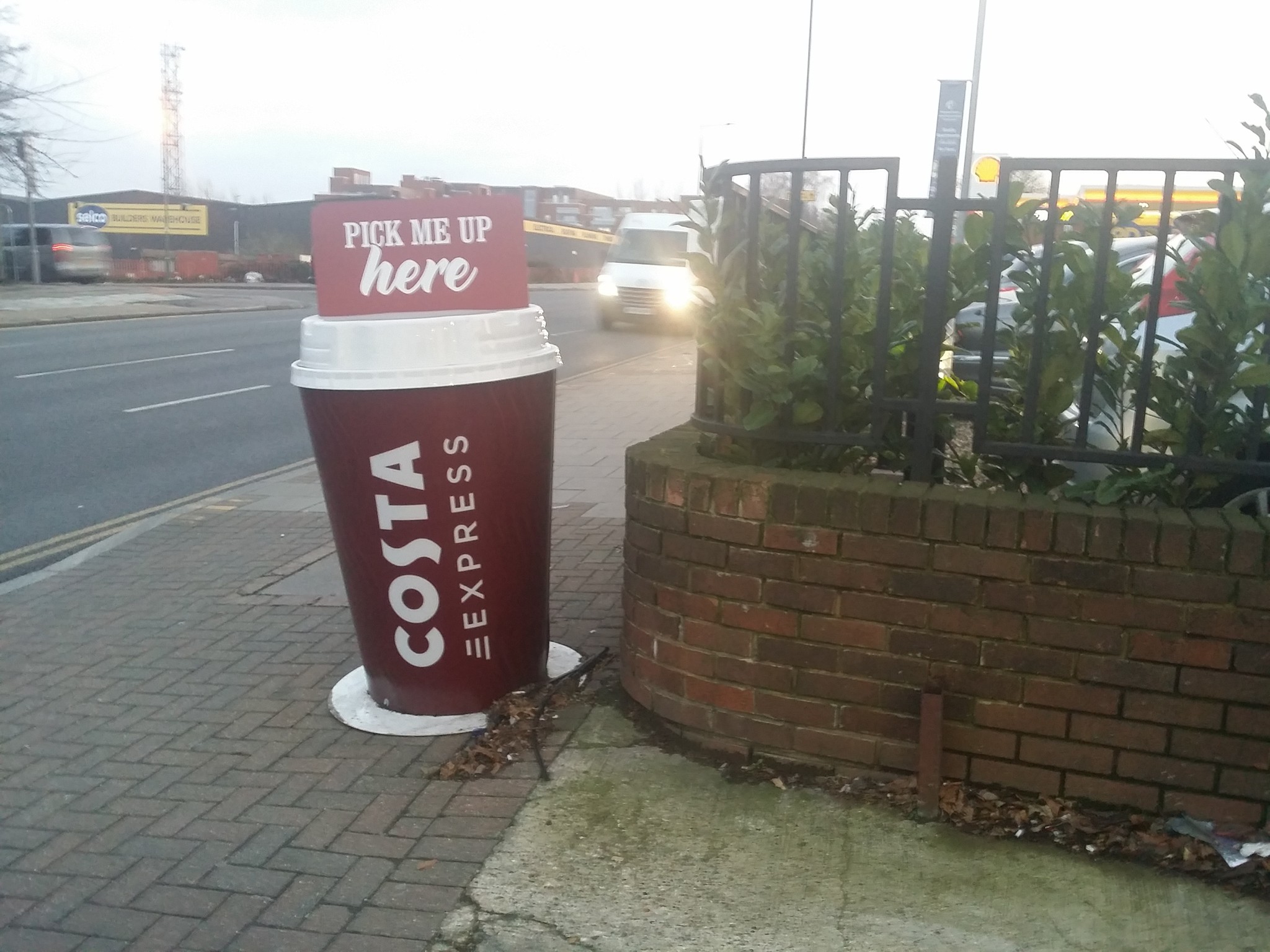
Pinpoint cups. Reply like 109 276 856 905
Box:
289 305 565 716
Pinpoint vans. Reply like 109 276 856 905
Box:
0 222 114 284
596 211 718 333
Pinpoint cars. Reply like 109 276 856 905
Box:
951 198 1269 487
